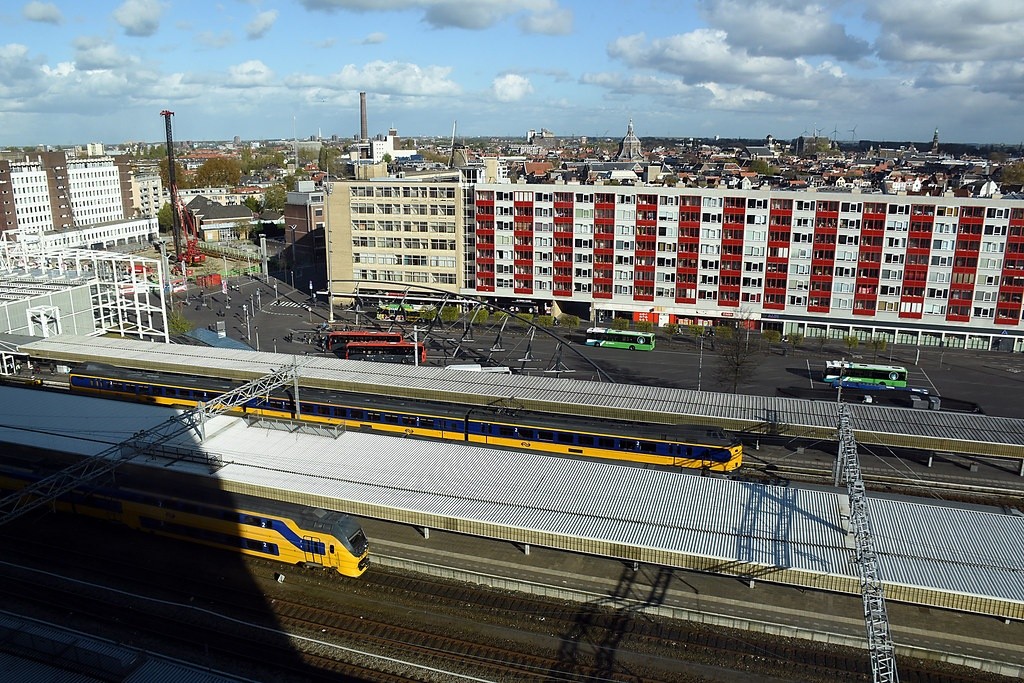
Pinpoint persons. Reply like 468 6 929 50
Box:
553 316 559 325
201 284 344 344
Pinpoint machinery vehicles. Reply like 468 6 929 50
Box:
172 183 206 265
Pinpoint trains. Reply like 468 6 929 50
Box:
66 360 743 477
1 453 371 579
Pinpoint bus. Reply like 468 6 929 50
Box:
344 339 428 365
326 331 403 354
823 359 909 391
585 326 656 352
